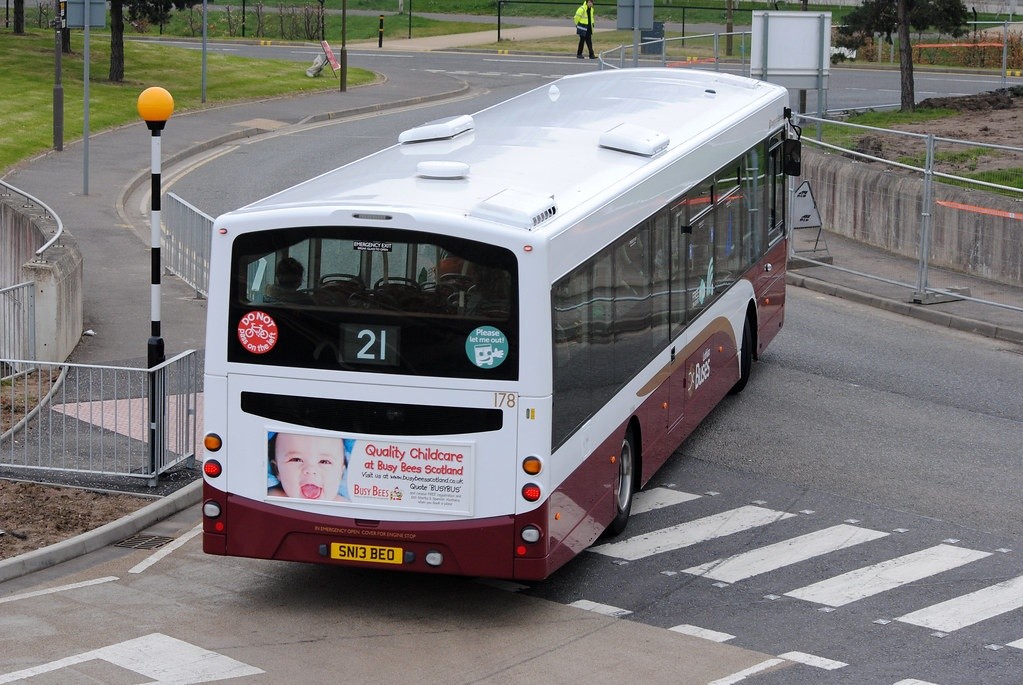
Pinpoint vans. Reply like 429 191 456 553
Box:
830 24 857 64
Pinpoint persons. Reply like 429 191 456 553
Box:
786 146 800 175
573 0 595 59
268 432 350 502
264 259 316 304
433 254 510 311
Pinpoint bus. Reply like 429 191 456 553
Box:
201 67 804 583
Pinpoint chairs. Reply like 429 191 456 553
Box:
262 256 513 319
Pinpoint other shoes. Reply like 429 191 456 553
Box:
577 55 584 59
589 57 598 59
